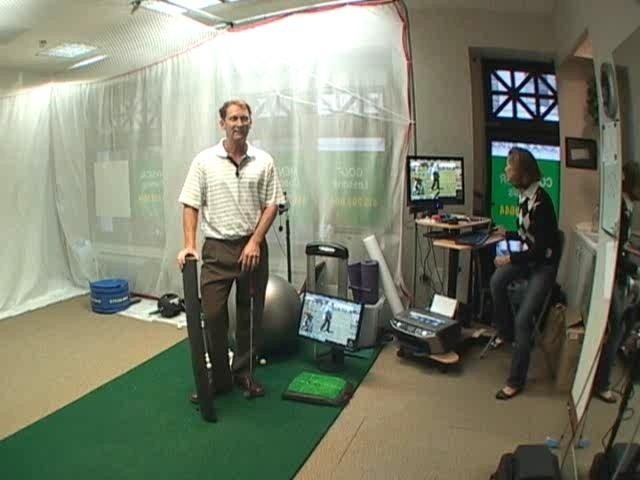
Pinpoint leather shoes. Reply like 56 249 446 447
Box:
233 374 264 398
191 378 232 403
495 389 520 399
488 338 504 350
595 390 616 402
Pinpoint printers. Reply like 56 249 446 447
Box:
389 292 462 355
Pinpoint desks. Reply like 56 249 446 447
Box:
398 214 504 372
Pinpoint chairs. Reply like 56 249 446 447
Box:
479 231 564 381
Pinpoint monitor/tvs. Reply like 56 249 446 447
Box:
405 154 465 218
296 290 365 374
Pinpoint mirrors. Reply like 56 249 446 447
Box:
563 31 640 478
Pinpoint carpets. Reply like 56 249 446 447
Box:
0 330 395 479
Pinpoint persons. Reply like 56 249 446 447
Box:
175 98 288 406
319 302 334 333
430 162 442 190
299 311 314 338
490 146 560 401
411 176 425 196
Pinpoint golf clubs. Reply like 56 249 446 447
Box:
243 275 256 400
545 323 611 450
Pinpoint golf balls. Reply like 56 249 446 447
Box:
204 348 234 369
260 359 266 364
177 324 183 329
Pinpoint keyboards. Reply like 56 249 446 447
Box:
456 231 489 246
